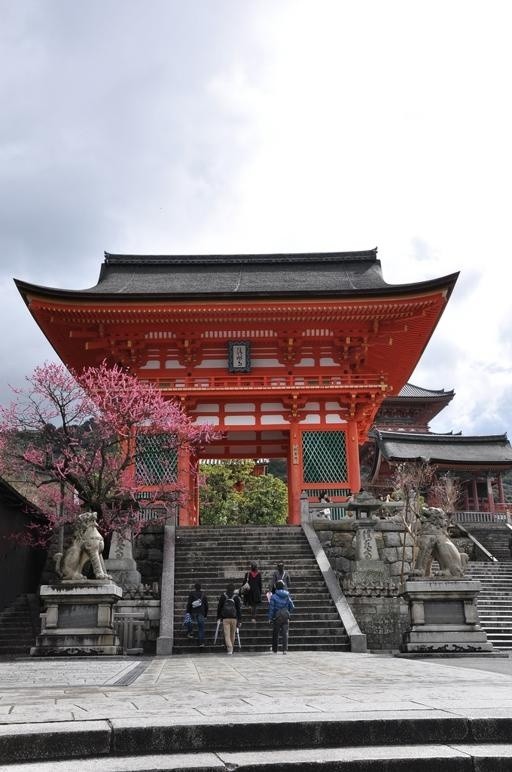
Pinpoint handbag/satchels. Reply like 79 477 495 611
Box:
241 583 249 595
275 580 286 587
274 609 290 624
192 599 202 608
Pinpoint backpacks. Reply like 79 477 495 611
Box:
220 592 236 617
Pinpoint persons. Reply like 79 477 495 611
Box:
185 583 208 647
267 580 295 654
318 492 333 521
269 560 292 621
241 561 263 623
215 584 244 655
376 493 390 518
345 492 357 519
